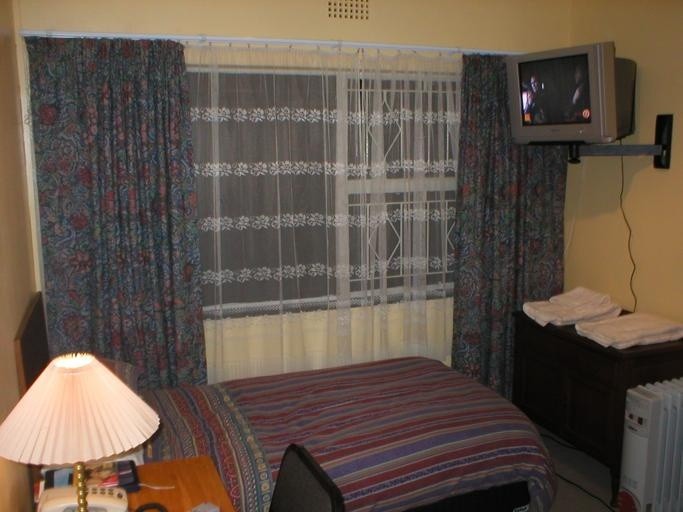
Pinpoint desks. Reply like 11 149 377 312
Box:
126 452 235 512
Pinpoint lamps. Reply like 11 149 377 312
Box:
0 348 160 512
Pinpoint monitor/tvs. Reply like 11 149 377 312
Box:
504 41 636 145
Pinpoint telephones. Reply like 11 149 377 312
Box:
37 484 129 512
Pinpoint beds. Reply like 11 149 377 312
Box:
15 289 552 512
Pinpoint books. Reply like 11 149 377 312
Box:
34 460 118 502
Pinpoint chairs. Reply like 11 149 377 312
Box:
265 442 348 512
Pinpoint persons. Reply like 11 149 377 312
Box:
568 64 588 121
522 72 552 126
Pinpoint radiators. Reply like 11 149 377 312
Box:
616 374 680 511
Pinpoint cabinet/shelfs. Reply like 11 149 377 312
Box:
507 304 682 510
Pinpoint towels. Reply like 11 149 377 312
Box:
520 285 682 352
